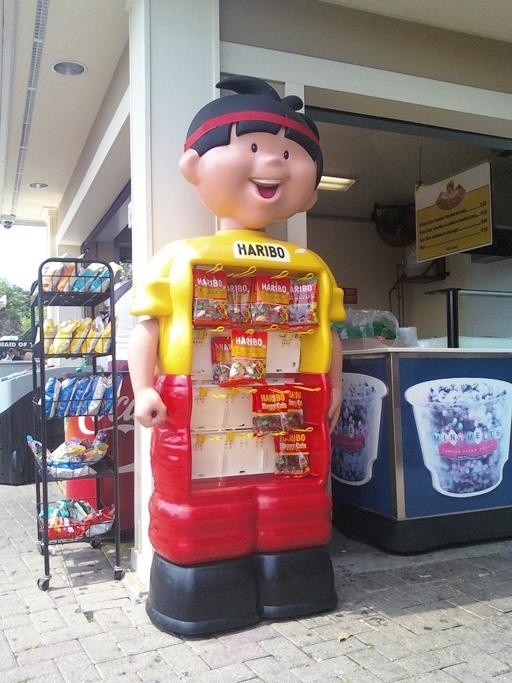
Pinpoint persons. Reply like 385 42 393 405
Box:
1 345 32 362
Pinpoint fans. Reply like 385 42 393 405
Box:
376 206 416 247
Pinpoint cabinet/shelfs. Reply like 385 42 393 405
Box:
29 257 125 591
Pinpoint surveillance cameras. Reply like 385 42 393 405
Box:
2 216 14 225
2 222 13 229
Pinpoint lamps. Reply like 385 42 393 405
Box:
316 176 356 192
